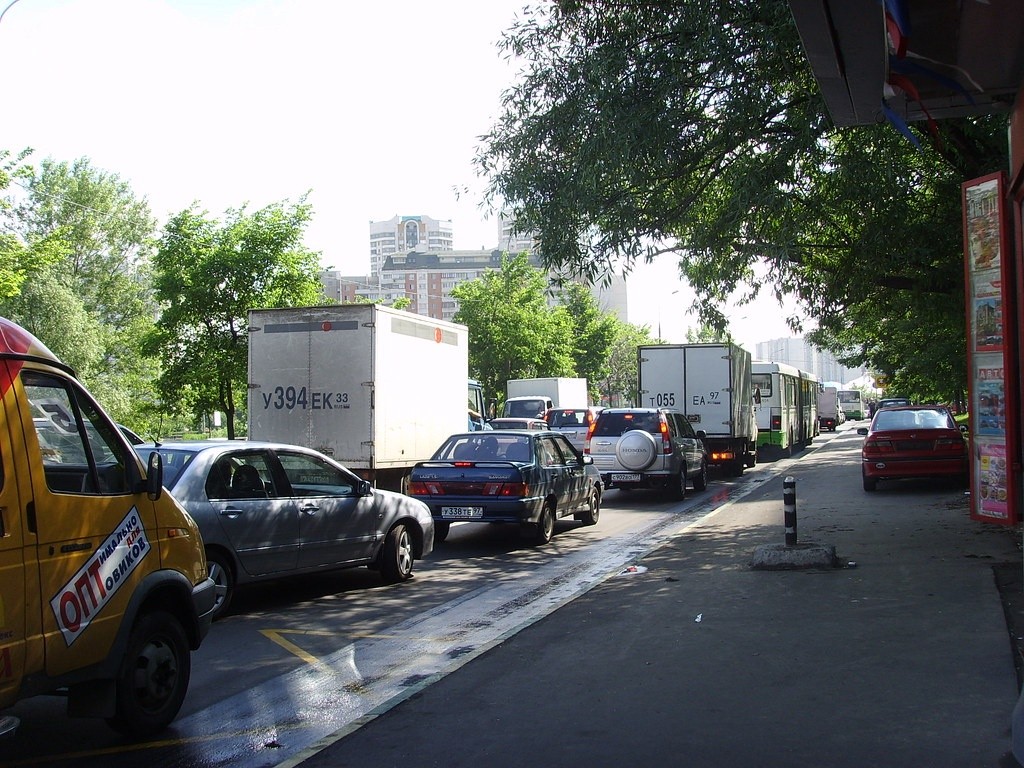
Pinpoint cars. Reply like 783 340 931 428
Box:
100 439 435 623
32 416 147 462
855 406 972 491
407 429 604 545
838 405 846 425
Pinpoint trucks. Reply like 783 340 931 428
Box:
818 386 840 432
502 377 587 422
636 342 761 478
247 303 497 498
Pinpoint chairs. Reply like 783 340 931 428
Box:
232 465 269 498
161 464 179 486
506 442 531 461
453 442 495 458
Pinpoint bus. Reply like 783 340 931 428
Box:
837 390 865 421
751 360 825 457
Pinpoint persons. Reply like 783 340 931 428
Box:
868 398 875 422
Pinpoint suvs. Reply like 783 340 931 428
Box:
476 416 551 459
544 404 605 458
875 398 911 416
584 407 707 500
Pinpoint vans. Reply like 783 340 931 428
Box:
0 316 228 744
